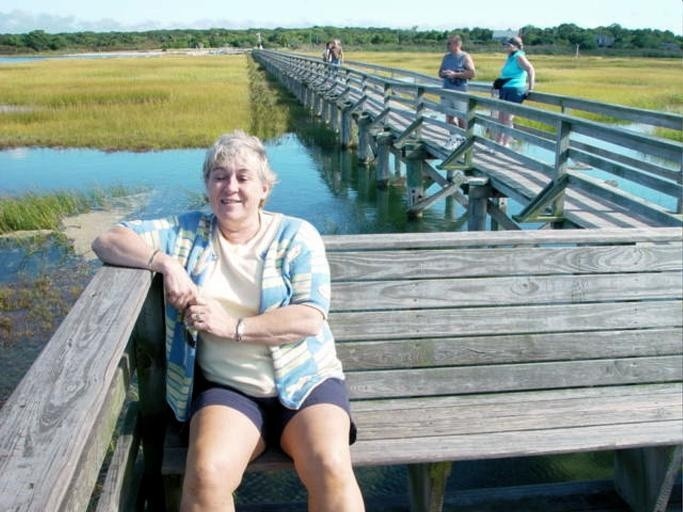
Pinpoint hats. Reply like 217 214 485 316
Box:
504 35 524 49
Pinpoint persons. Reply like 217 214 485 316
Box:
491 35 534 150
89 129 370 512
322 41 332 62
437 34 476 129
325 38 344 73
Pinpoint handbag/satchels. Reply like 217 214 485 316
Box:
493 77 513 89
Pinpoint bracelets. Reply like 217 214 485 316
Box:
527 89 533 90
146 246 160 271
232 317 244 344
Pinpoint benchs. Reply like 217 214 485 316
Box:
159 225 682 510
